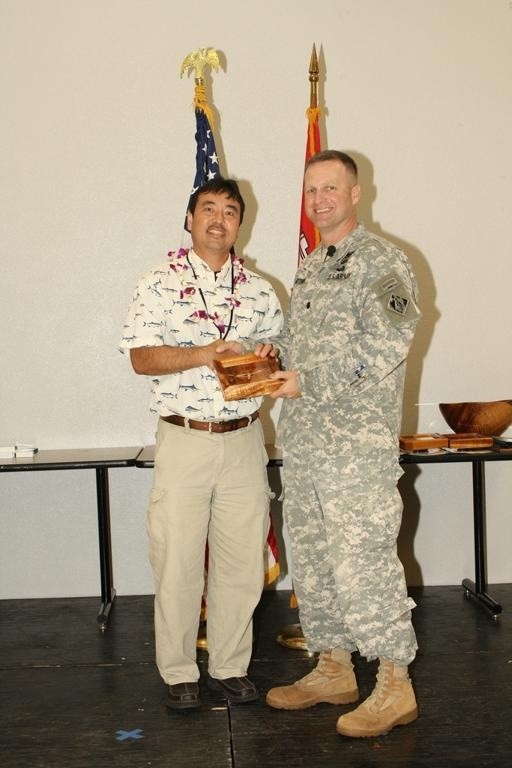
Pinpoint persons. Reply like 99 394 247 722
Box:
118 176 285 710
215 149 421 739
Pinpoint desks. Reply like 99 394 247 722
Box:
1 434 141 623
138 441 509 613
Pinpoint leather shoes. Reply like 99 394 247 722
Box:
166 683 201 709
206 673 259 703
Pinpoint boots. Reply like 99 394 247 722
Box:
336 655 418 738
266 648 359 711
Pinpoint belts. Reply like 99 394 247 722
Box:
159 410 259 433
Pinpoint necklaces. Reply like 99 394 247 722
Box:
168 248 246 339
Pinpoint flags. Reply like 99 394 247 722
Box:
297 110 327 266
184 114 235 254
